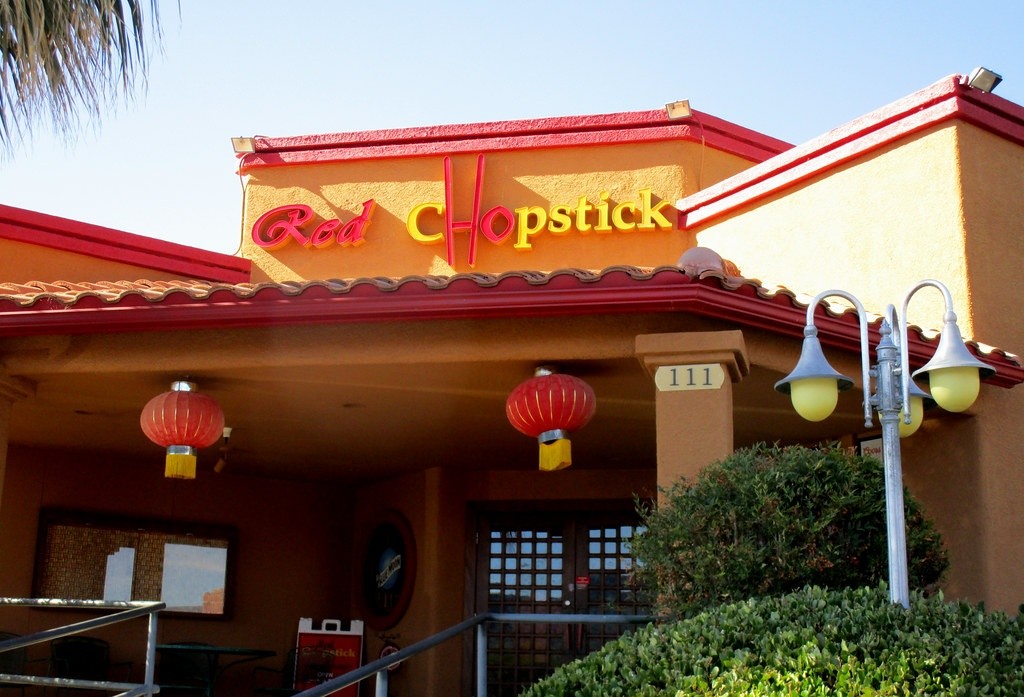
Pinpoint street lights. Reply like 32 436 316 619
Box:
771 278 998 608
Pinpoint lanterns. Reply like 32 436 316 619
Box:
504 363 598 472
138 379 228 476
